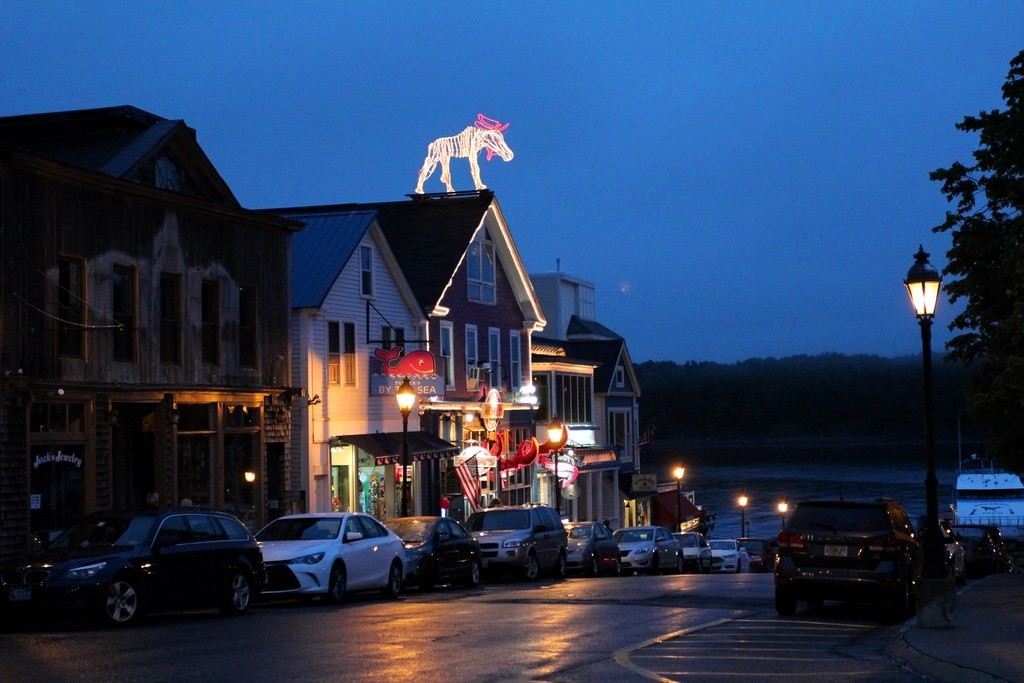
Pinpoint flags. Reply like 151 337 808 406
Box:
454 456 482 511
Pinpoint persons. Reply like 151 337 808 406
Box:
488 499 501 508
603 519 614 534
145 491 160 505
181 499 192 507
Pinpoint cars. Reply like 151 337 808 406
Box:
671 532 713 574
950 524 1008 577
382 516 483 596
908 514 972 597
562 521 621 577
612 526 684 576
253 511 409 606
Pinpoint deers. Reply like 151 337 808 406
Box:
414 112 515 194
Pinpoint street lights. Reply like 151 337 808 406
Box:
394 374 417 516
778 500 788 528
674 466 684 532
903 245 960 628
547 419 564 516
738 495 747 538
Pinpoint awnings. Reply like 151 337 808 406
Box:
337 431 459 465
650 490 702 527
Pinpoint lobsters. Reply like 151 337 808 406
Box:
490 424 568 471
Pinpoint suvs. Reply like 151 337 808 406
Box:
777 496 922 626
463 503 568 583
0 505 268 631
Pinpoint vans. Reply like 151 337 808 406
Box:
706 540 751 573
736 537 770 573
